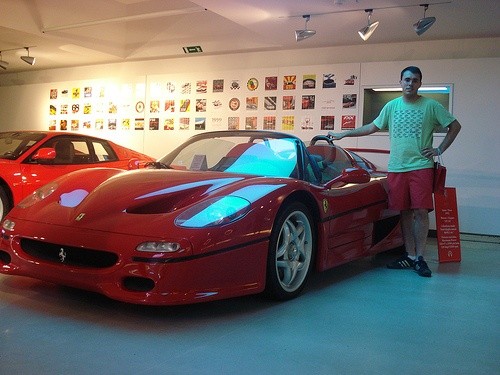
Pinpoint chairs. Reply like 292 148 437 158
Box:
227 135 351 171
54 142 74 163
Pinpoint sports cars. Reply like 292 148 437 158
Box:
0 129 187 224
1 130 430 307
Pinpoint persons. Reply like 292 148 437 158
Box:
328 66 461 277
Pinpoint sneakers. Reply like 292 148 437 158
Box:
413 255 431 277
387 252 415 269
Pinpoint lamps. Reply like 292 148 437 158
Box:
0 51 9 71
413 4 436 36
295 15 317 42
357 9 380 41
20 47 35 65
